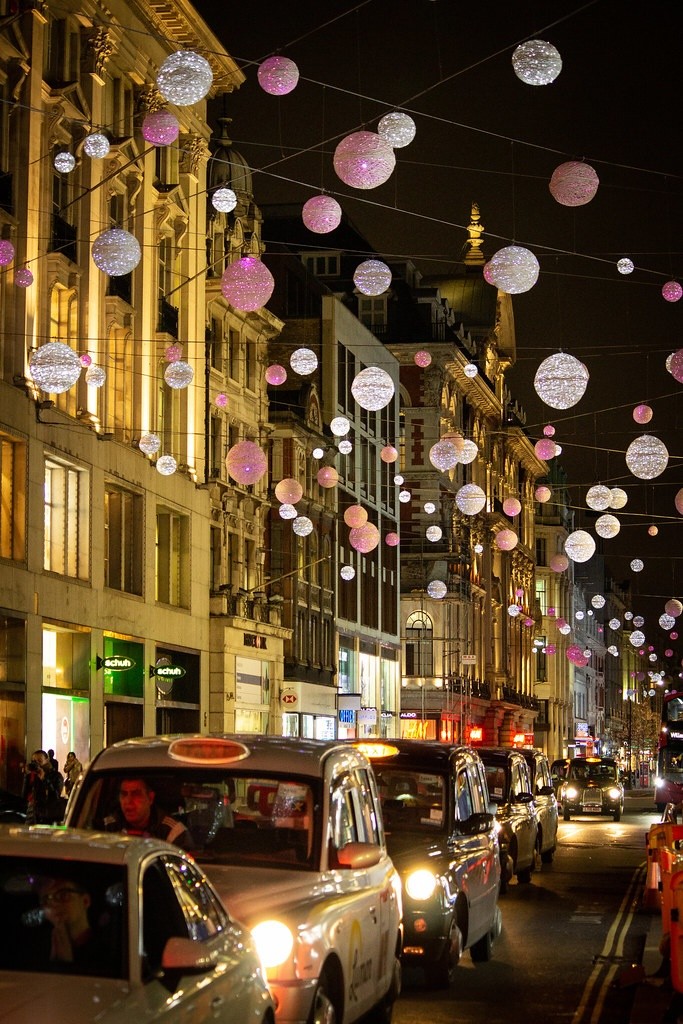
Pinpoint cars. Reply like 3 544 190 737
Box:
0 825 276 1024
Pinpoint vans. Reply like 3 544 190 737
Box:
521 750 557 863
66 733 406 1024
360 742 503 989
550 759 569 784
479 747 538 890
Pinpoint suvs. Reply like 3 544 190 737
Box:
561 758 625 822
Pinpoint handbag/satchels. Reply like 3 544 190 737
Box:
64 778 71 789
33 796 68 822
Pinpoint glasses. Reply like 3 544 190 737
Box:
41 888 81 906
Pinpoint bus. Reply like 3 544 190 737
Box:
653 691 683 812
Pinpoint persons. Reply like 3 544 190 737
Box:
95 773 195 852
21 750 64 825
575 767 589 779
47 749 59 772
63 751 84 797
30 869 154 985
558 766 565 777
